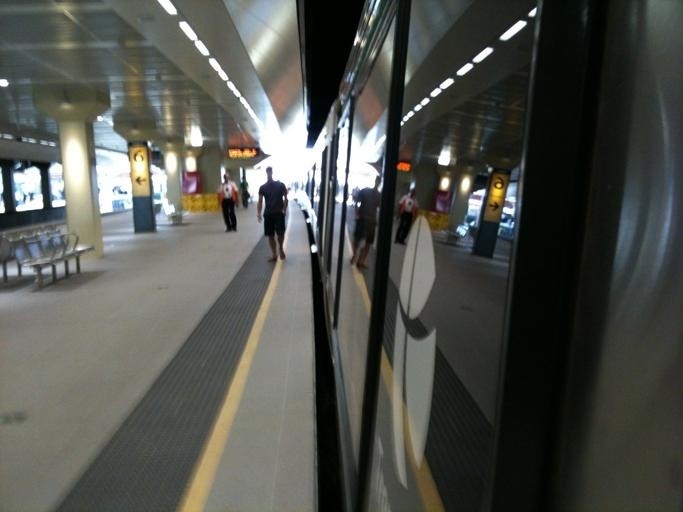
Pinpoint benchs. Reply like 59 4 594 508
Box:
0 223 92 291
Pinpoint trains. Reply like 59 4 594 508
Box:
292 0 683 512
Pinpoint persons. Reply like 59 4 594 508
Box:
216 173 241 233
256 166 288 262
238 176 249 207
349 175 381 269
393 186 419 246
